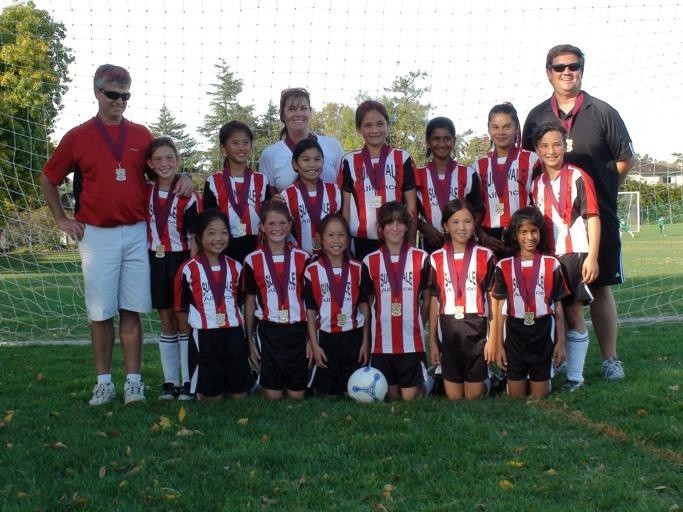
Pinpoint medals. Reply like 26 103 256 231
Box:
277 308 289 324
115 168 128 182
453 305 466 321
563 138 574 154
337 313 347 327
524 310 534 326
391 301 403 318
155 243 167 259
559 222 569 238
214 313 226 327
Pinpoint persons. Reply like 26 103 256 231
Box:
203 86 545 303
429 198 498 401
40 63 155 408
492 206 571 398
362 200 429 403
531 120 602 393
142 137 202 403
618 213 634 241
173 211 251 402
244 200 313 399
524 44 634 381
656 215 669 238
302 212 370 396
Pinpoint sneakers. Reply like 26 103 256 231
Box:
179 382 194 401
601 356 625 380
159 383 179 401
124 379 146 405
89 383 116 406
558 379 583 392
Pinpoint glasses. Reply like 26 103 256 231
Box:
281 88 306 95
100 89 130 100
549 62 582 72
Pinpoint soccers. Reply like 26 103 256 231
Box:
347 368 386 405
